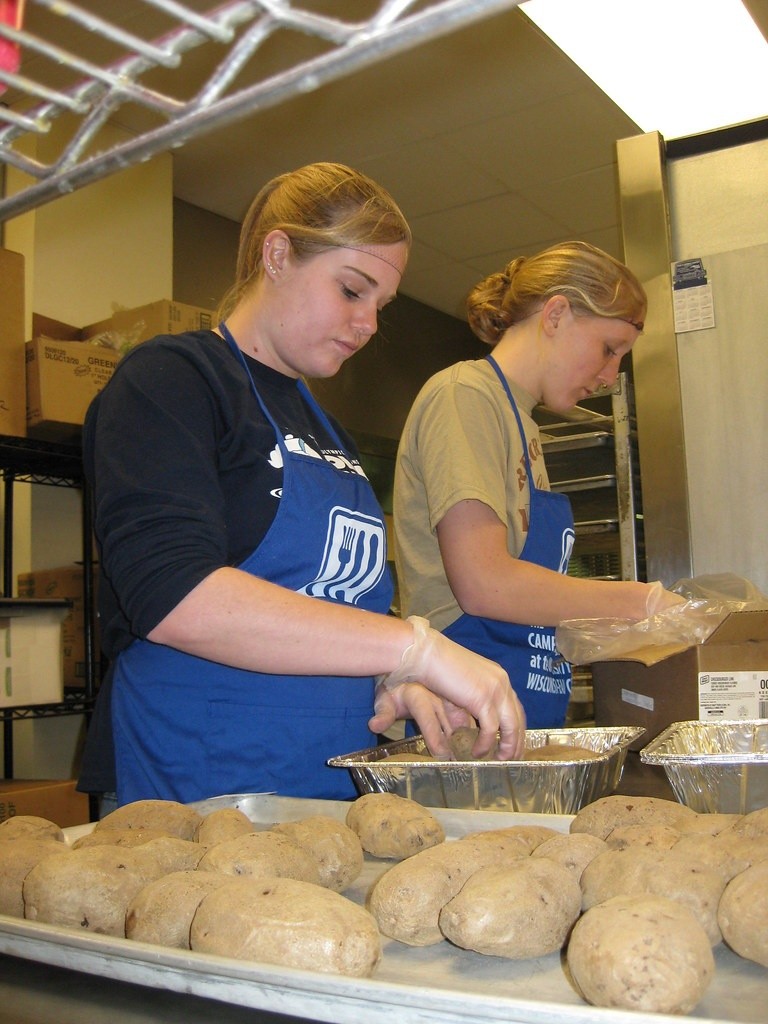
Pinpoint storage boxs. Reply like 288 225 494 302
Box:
641 719 767 815
589 609 767 752
28 301 236 424
0 470 90 829
328 724 645 815
1 246 25 435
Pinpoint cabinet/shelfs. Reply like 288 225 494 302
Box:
0 439 96 835
528 373 639 725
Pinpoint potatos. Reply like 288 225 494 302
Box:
0 726 768 1022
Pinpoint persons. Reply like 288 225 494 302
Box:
75 161 527 810
389 238 692 739
